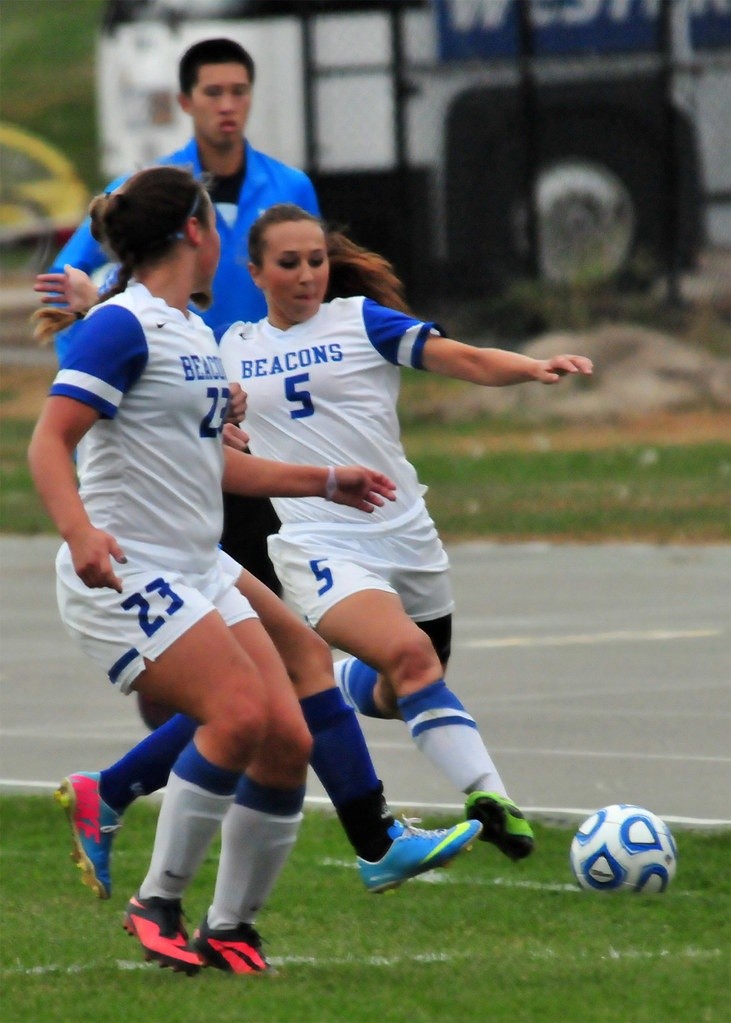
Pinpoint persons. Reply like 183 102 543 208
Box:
48 37 325 722
32 204 595 859
27 166 312 973
55 192 485 899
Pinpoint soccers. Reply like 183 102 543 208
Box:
571 804 678 896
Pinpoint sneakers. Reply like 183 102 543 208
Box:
464 790 535 861
121 895 203 981
54 772 120 901
193 916 281 985
354 818 486 895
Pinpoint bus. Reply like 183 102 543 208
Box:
90 0 730 349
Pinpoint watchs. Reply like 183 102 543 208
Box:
324 465 337 500
